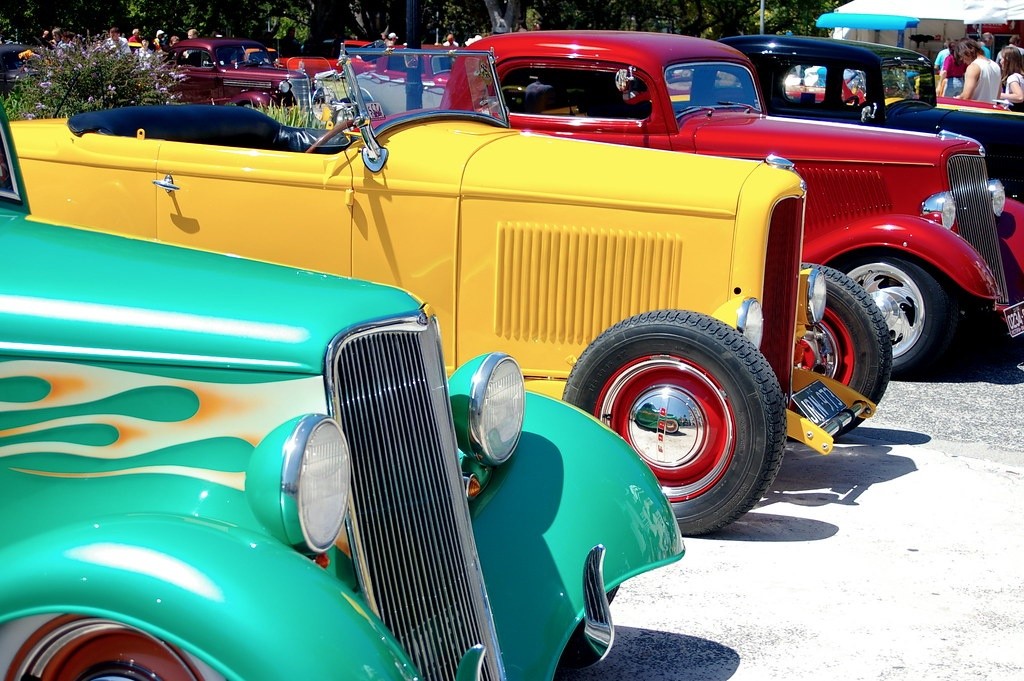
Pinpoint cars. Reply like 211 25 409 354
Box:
0 212 688 681
276 39 462 118
0 50 892 543
688 35 1024 213
344 29 1024 382
143 38 310 115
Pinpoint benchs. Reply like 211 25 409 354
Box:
68 105 348 155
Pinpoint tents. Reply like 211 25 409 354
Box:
833 0 1024 61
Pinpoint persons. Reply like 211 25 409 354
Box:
934 32 1024 112
41 23 540 69
